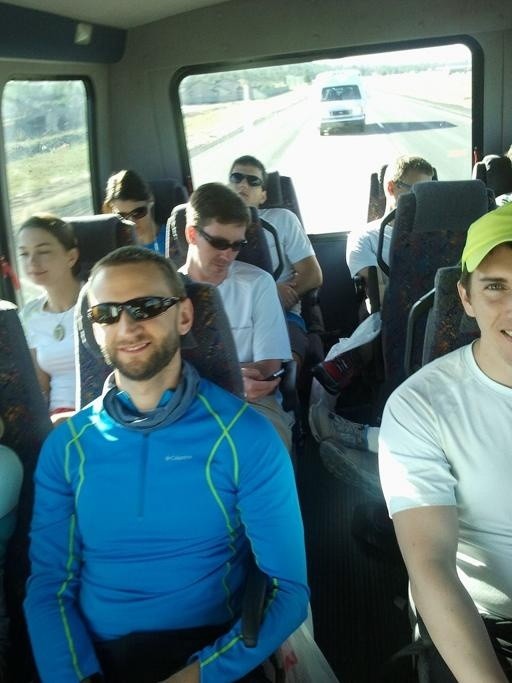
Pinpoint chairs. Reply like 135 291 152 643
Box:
377 180 497 397
352 163 390 297
259 172 304 229
0 299 48 589
403 267 481 381
146 177 189 227
166 203 312 440
74 274 246 414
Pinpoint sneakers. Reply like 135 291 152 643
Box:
308 401 387 507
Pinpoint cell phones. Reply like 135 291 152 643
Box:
265 368 284 380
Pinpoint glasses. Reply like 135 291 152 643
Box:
116 201 150 221
191 223 249 252
228 172 266 192
87 294 182 325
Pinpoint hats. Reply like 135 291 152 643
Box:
459 200 512 274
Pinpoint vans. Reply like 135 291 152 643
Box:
430 64 471 104
312 78 369 138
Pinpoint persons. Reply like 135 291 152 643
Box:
14 215 91 429
175 181 299 462
15 246 313 683
99 163 167 262
226 152 321 452
342 151 437 315
377 200 510 683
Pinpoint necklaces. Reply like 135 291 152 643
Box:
41 293 80 342
